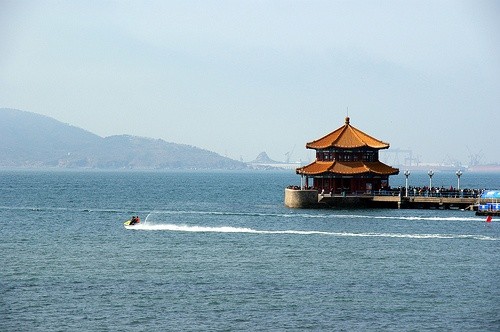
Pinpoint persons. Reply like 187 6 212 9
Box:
330 186 333 197
129 216 139 225
288 185 318 190
463 188 486 198
379 186 461 198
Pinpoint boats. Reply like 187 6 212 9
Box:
123 220 143 230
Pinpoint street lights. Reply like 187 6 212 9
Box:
456 169 464 189
427 170 434 191
403 169 410 197
